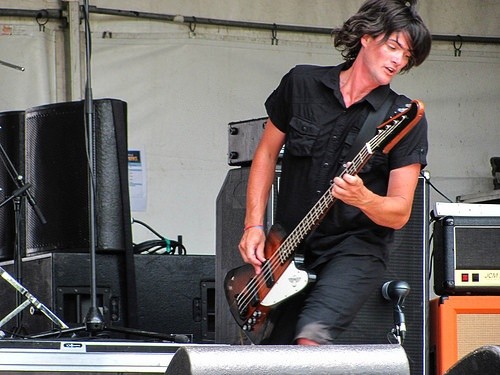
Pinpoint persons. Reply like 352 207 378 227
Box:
238 1 432 346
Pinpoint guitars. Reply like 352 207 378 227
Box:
219 98 427 347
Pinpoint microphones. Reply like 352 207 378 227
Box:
381 281 410 300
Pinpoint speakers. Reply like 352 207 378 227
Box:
431 295 500 375
0 99 134 260
0 251 127 344
216 166 430 375
132 253 215 344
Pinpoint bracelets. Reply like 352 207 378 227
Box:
243 225 265 230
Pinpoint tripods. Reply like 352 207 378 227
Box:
28 1 193 344
